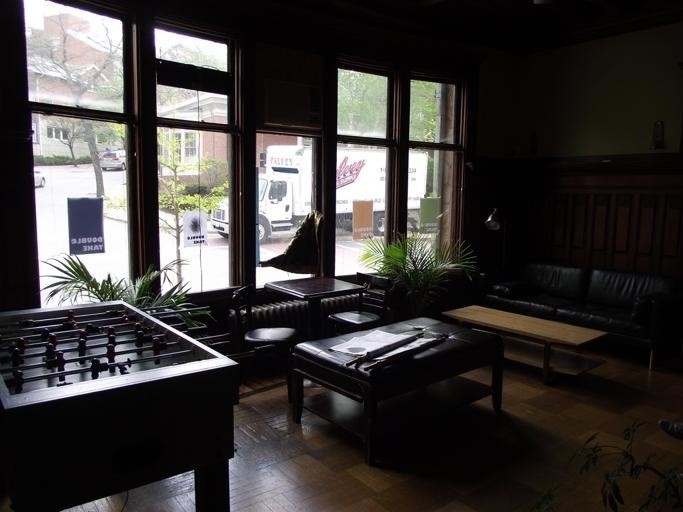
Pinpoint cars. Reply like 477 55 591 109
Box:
99 150 126 171
33 169 46 187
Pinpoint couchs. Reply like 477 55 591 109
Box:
490 260 683 373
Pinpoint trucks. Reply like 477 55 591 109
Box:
211 145 428 245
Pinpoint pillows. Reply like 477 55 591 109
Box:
523 263 592 301
630 293 661 320
585 269 657 309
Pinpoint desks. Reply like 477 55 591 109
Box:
289 315 504 455
264 277 365 339
442 305 607 383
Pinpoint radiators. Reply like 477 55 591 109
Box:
230 292 370 336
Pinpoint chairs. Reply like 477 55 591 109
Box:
232 284 296 403
328 273 393 331
